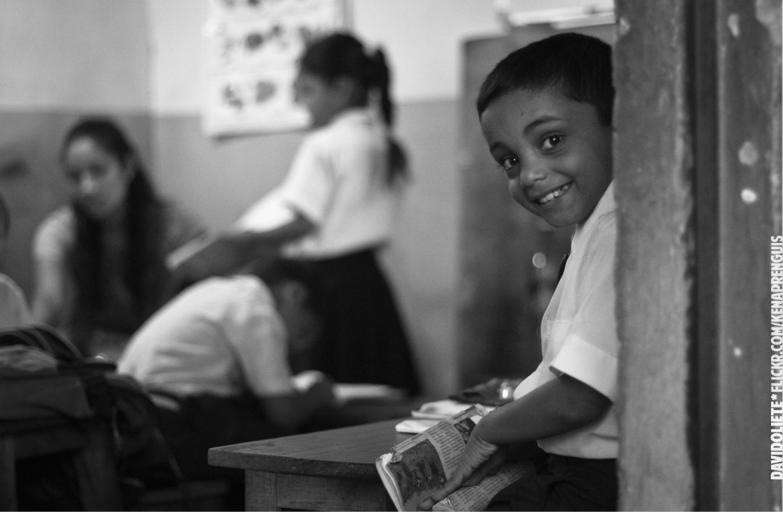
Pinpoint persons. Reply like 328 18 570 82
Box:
96 251 339 510
412 31 621 512
27 112 210 364
209 31 421 400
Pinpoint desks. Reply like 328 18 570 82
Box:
1 414 85 512
207 421 418 512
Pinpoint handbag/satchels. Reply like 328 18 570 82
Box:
0 324 110 435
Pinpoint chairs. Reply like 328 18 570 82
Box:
86 383 234 511
